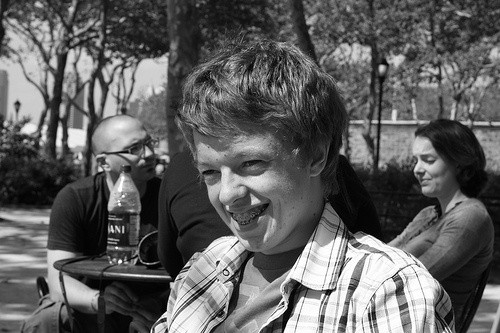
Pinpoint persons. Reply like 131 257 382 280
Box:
145 41 456 333
21 114 162 333
159 150 382 279
387 120 495 329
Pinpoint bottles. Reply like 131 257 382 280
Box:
106 165 142 267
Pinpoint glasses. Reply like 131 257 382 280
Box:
101 136 161 156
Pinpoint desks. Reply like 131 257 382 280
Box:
53 256 171 333
